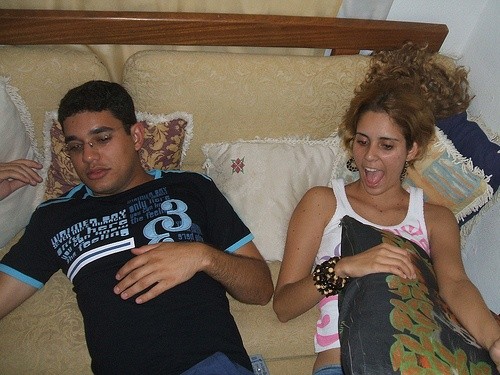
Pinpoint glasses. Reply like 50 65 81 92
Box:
60 126 121 159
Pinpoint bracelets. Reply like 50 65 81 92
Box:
313 257 350 298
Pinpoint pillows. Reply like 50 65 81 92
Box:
0 44 111 155
122 50 374 175
32 111 193 208
200 137 342 265
0 74 44 246
338 215 498 375
328 126 493 224
434 108 500 227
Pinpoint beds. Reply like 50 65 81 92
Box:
0 8 500 375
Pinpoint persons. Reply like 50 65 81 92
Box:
273 40 500 375
0 80 274 375
